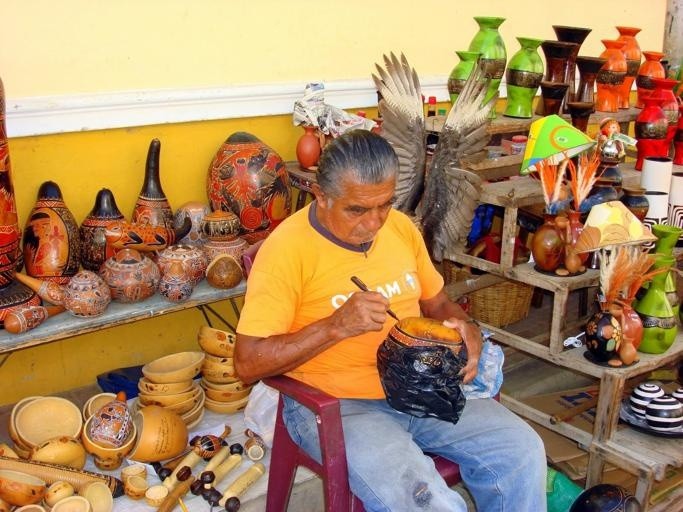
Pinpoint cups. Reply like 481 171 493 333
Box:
125 475 149 499
120 464 147 482
50 495 91 512
77 480 112 512
14 505 44 512
145 486 169 507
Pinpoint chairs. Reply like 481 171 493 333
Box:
242 237 499 512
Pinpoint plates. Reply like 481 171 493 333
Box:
618 394 683 439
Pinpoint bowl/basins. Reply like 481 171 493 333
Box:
197 325 253 414
84 393 117 422
9 396 86 470
136 350 206 430
0 469 45 507
83 395 94 423
84 411 137 461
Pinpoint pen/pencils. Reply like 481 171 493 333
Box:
350 276 401 323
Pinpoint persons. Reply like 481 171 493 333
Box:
232 128 547 512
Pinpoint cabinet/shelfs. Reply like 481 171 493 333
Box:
0 272 318 512
278 115 683 512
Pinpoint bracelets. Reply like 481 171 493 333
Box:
463 317 482 329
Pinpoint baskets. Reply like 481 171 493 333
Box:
440 236 537 327
